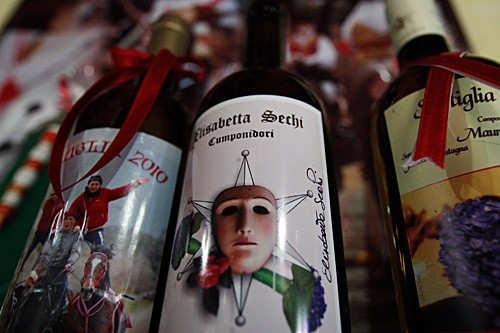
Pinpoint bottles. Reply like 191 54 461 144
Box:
156 0 353 333
0 12 193 333
369 0 499 332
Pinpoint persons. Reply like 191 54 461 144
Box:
39 211 82 318
15 192 64 282
67 175 149 250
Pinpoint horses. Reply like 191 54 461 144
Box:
6 243 127 333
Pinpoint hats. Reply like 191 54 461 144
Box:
65 210 78 219
88 176 102 185
